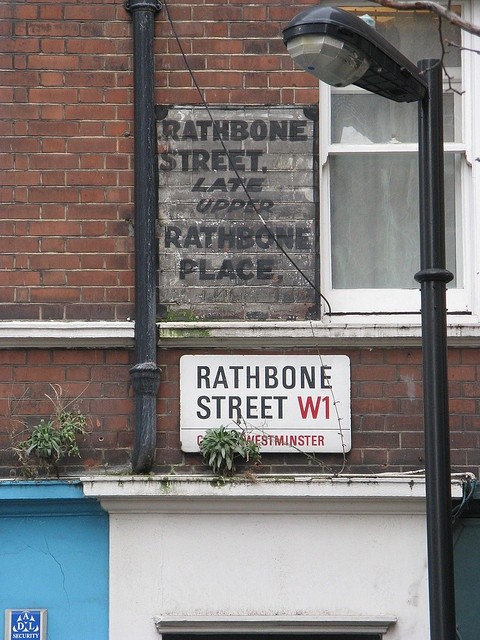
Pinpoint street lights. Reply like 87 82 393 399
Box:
283 5 454 640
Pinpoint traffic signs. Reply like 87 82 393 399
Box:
179 355 352 453
